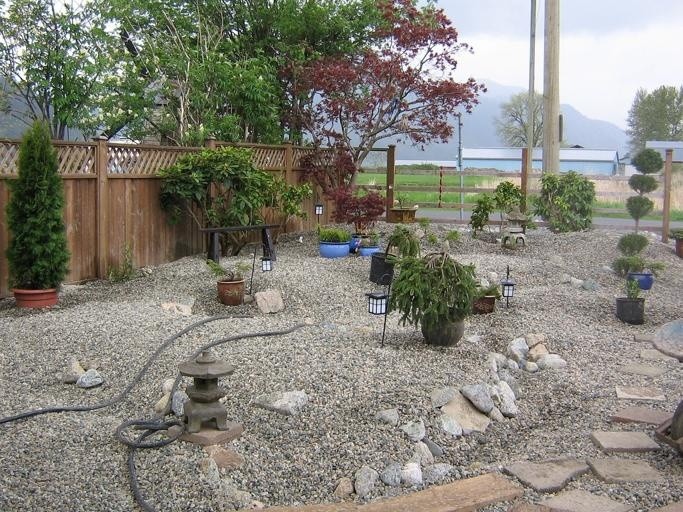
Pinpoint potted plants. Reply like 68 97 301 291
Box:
670 229 682 256
617 278 644 325
209 258 255 304
355 230 382 257
318 228 353 256
389 190 416 223
2 117 75 307
390 252 502 346
611 256 666 290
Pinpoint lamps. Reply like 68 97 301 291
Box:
503 282 516 308
364 292 390 315
